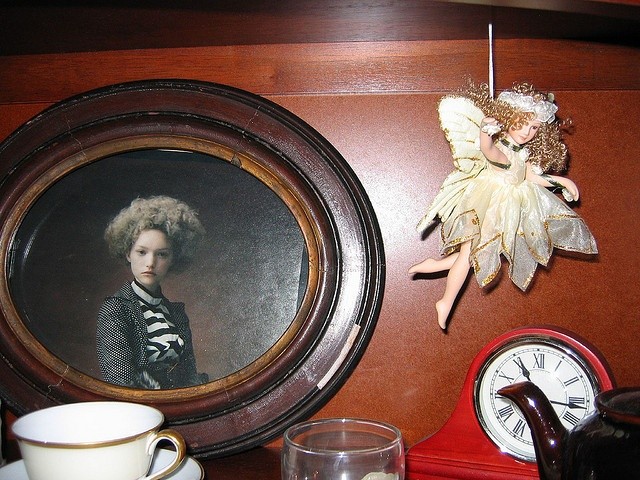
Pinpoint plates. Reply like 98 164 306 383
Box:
0 447 205 480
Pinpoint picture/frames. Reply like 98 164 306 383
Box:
0 79 385 459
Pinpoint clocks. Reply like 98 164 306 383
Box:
406 324 615 480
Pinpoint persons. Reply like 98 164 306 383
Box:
407 82 599 331
96 195 210 388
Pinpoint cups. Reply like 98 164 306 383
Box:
12 400 186 480
281 417 405 479
